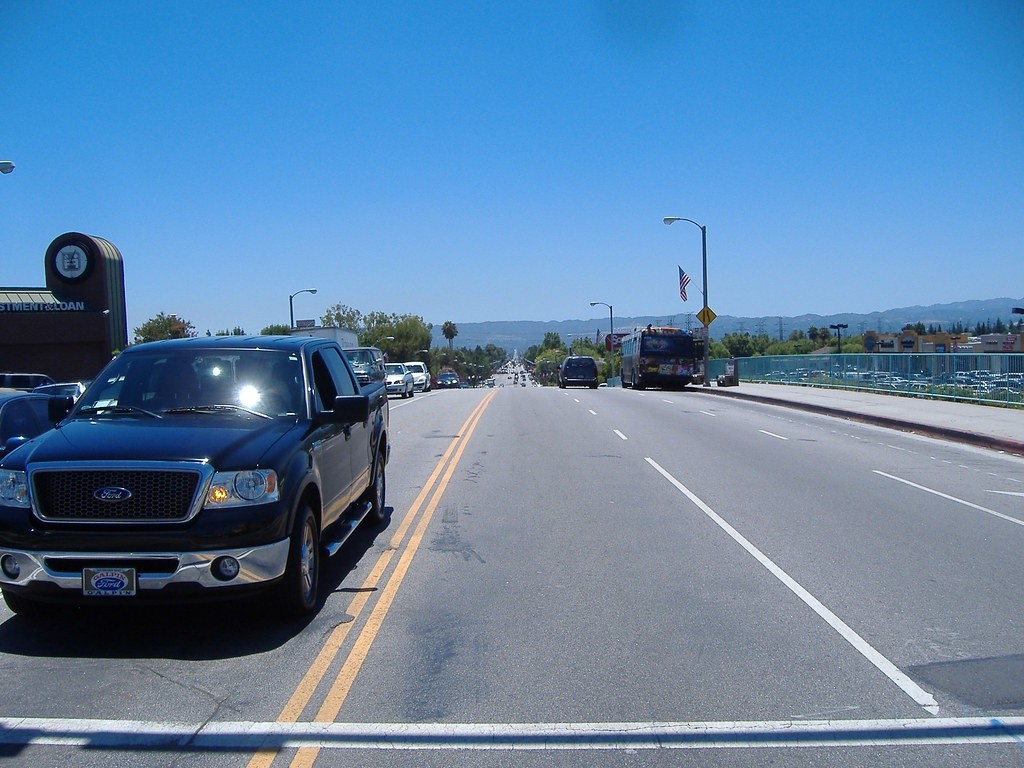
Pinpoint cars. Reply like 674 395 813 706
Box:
434 373 461 389
460 381 470 388
764 371 1024 403
472 360 538 389
0 365 159 455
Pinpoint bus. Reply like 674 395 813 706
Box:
617 323 695 390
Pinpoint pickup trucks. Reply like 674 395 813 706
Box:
0 334 390 619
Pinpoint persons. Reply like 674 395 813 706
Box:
234 355 293 411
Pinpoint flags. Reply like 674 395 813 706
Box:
679 267 691 302
596 329 604 344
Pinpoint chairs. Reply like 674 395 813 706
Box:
134 360 201 414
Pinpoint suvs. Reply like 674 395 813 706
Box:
396 361 432 392
557 356 599 389
384 362 415 398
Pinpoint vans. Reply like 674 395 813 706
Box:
341 346 386 386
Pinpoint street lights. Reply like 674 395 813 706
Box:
830 324 848 354
663 216 712 387
590 302 616 380
290 289 317 328
567 333 583 356
